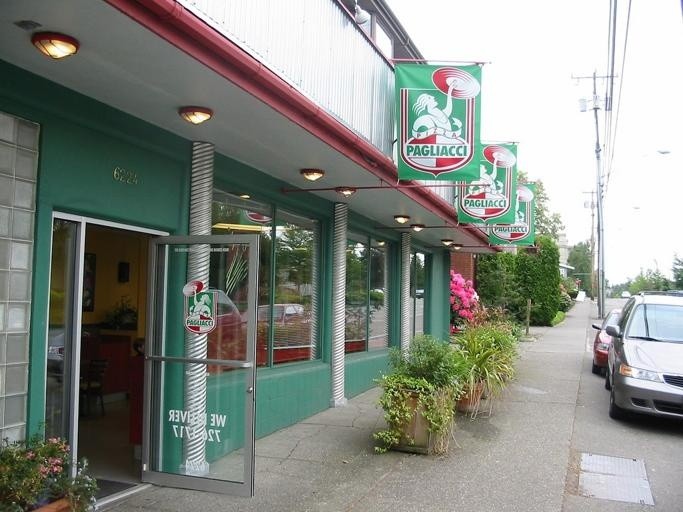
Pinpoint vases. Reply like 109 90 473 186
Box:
31 498 71 512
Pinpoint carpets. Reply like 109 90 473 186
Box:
95 478 137 499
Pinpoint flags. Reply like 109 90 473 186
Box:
487 182 534 247
456 141 518 226
391 62 481 186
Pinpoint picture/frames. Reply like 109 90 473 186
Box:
83 252 97 312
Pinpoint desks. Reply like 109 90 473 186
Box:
81 334 131 395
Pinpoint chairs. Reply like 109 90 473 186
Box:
79 359 105 417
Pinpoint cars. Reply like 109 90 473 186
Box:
245 302 310 343
589 285 683 426
45 328 68 383
367 285 423 303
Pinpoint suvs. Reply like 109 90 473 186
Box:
206 290 244 362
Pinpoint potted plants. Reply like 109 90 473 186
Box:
373 326 512 454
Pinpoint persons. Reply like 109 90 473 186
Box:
132 337 145 357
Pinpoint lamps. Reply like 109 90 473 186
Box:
300 168 324 182
31 32 79 59
441 239 453 245
354 4 370 24
178 106 212 124
453 244 463 249
335 187 356 196
410 224 425 231
394 215 410 224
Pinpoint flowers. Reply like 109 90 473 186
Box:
0 418 98 512
